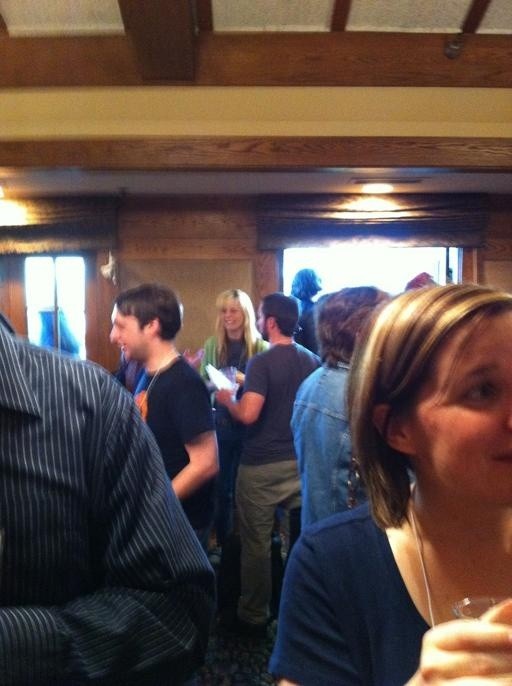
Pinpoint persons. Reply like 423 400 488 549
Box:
264 278 512 684
106 264 441 632
0 306 221 684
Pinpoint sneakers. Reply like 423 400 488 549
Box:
215 611 267 639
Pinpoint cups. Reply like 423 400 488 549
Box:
219 366 237 402
454 595 512 626
